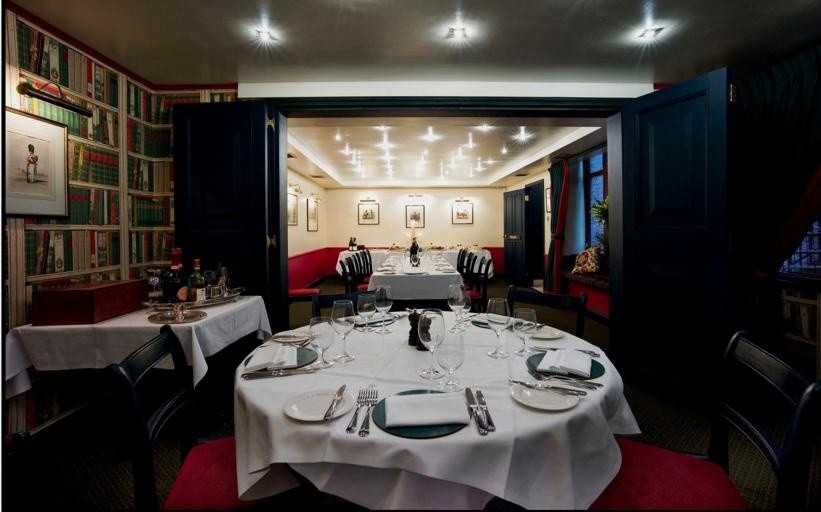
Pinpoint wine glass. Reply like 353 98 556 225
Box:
483 296 510 359
510 307 536 357
413 283 472 395
308 284 394 371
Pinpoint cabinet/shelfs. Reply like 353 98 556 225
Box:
2 1 241 445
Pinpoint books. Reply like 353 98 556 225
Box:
179 90 239 106
67 140 174 226
16 18 174 157
25 217 180 285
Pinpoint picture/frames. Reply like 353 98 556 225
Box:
287 191 299 225
358 201 380 225
4 104 70 217
306 197 319 232
451 200 474 225
406 204 425 229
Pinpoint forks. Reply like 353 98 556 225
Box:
358 390 378 437
346 389 369 432
528 369 603 391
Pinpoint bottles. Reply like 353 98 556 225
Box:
409 238 419 265
349 237 357 251
142 247 230 305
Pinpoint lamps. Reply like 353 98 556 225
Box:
639 2 665 38
441 0 470 41
254 1 281 43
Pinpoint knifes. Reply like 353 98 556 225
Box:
475 390 496 432
509 379 586 396
323 383 347 422
239 326 320 379
528 345 600 357
463 387 488 435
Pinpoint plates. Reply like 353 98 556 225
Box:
508 382 580 411
372 389 473 439
471 316 510 329
518 323 565 340
280 387 356 423
524 351 605 379
405 307 436 319
377 249 455 274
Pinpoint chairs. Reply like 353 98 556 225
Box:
308 291 376 323
372 248 461 301
339 249 373 316
16 295 258 512
590 330 819 512
100 323 266 512
457 247 493 313
503 283 588 339
